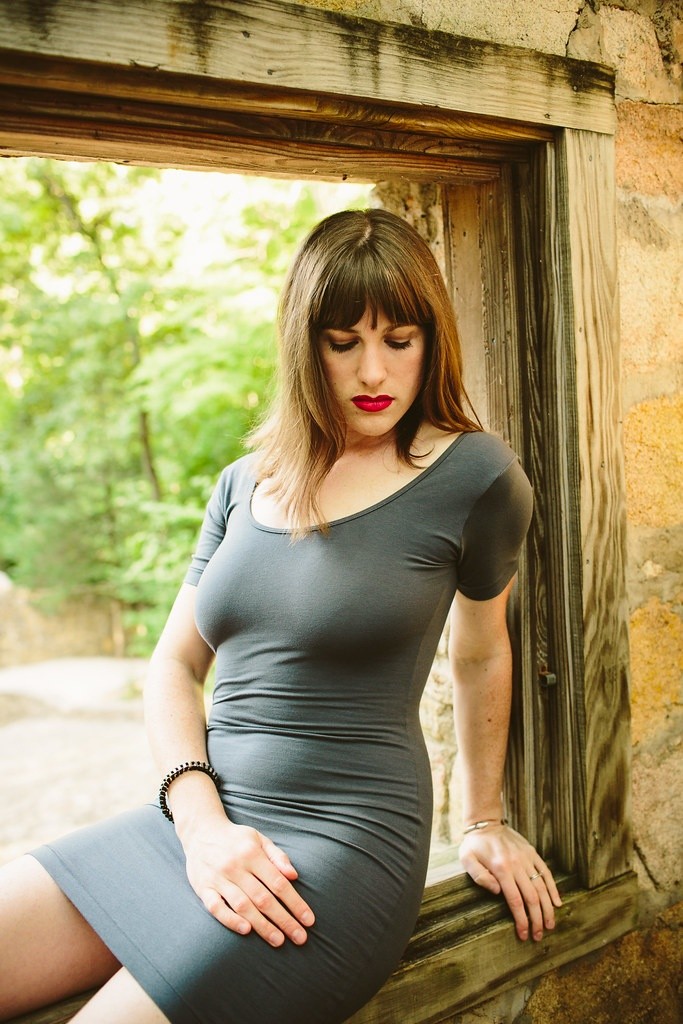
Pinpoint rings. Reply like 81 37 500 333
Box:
530 872 541 879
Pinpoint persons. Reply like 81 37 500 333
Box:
0 208 563 1024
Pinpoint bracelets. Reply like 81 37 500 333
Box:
461 817 511 834
158 761 223 824
